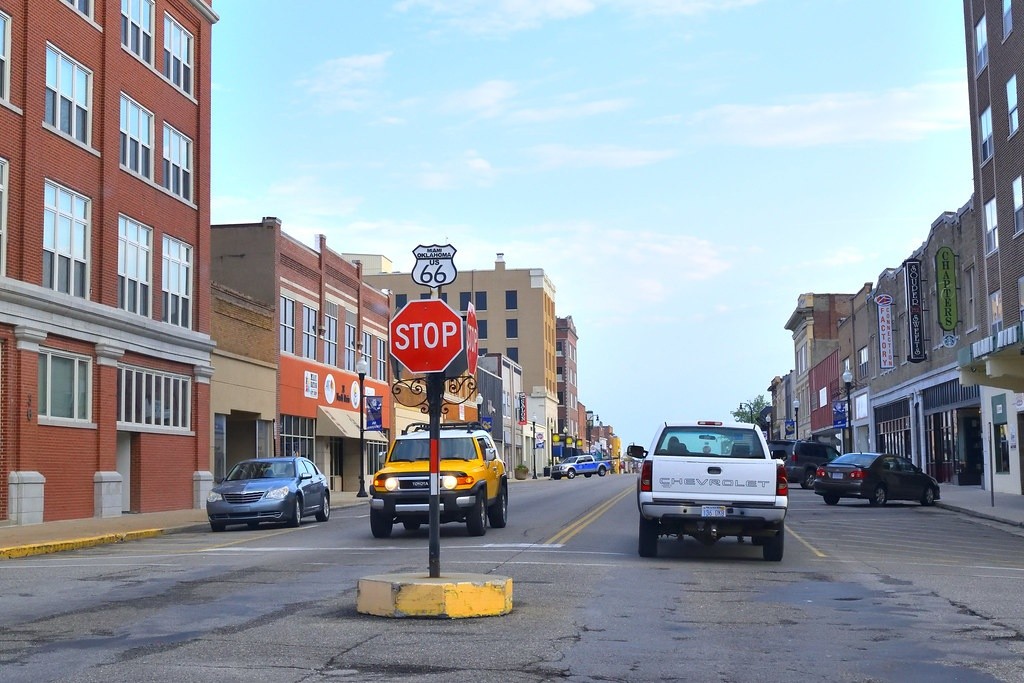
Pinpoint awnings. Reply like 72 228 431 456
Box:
315 405 389 443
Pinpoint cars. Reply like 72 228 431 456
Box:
205 456 332 529
814 452 942 506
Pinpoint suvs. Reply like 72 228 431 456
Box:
368 419 508 538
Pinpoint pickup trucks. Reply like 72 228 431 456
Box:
550 454 611 481
624 421 789 562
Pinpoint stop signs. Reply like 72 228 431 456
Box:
387 299 465 374
466 302 481 376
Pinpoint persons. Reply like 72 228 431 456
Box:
285 464 294 475
253 469 264 477
265 468 273 477
667 436 679 449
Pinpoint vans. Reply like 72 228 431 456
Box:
767 439 843 491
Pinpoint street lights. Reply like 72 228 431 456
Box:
738 402 753 423
474 392 484 422
531 412 537 479
793 397 800 440
356 355 370 500
766 414 770 441
564 426 567 459
842 369 853 453
